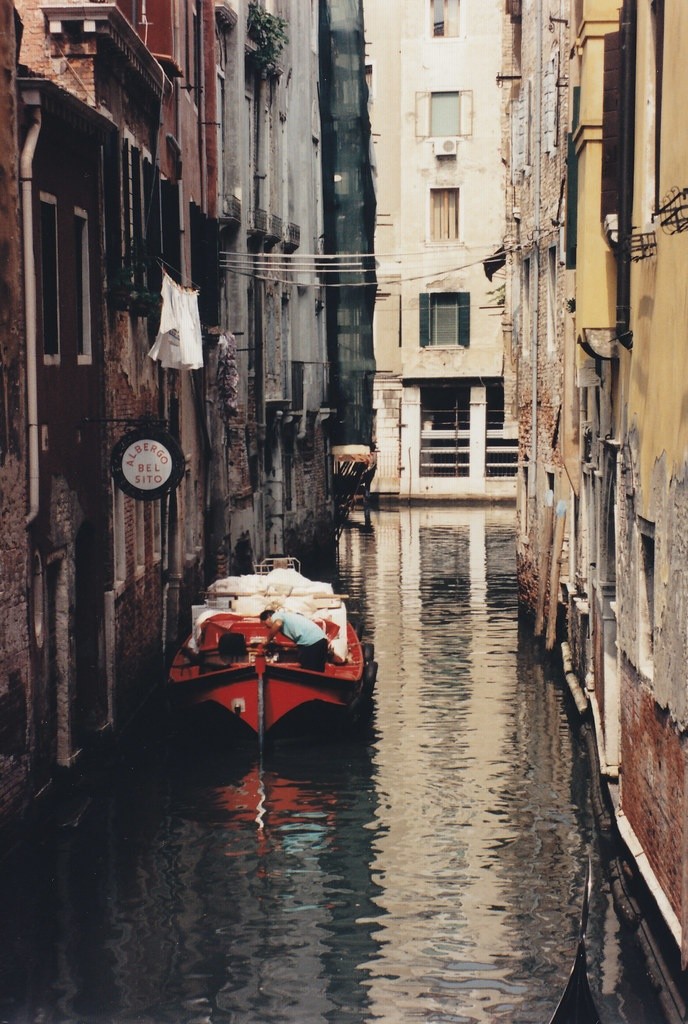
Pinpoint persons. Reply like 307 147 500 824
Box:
256 609 329 674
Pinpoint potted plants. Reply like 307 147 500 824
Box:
109 267 160 318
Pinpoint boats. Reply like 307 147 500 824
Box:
163 572 376 743
